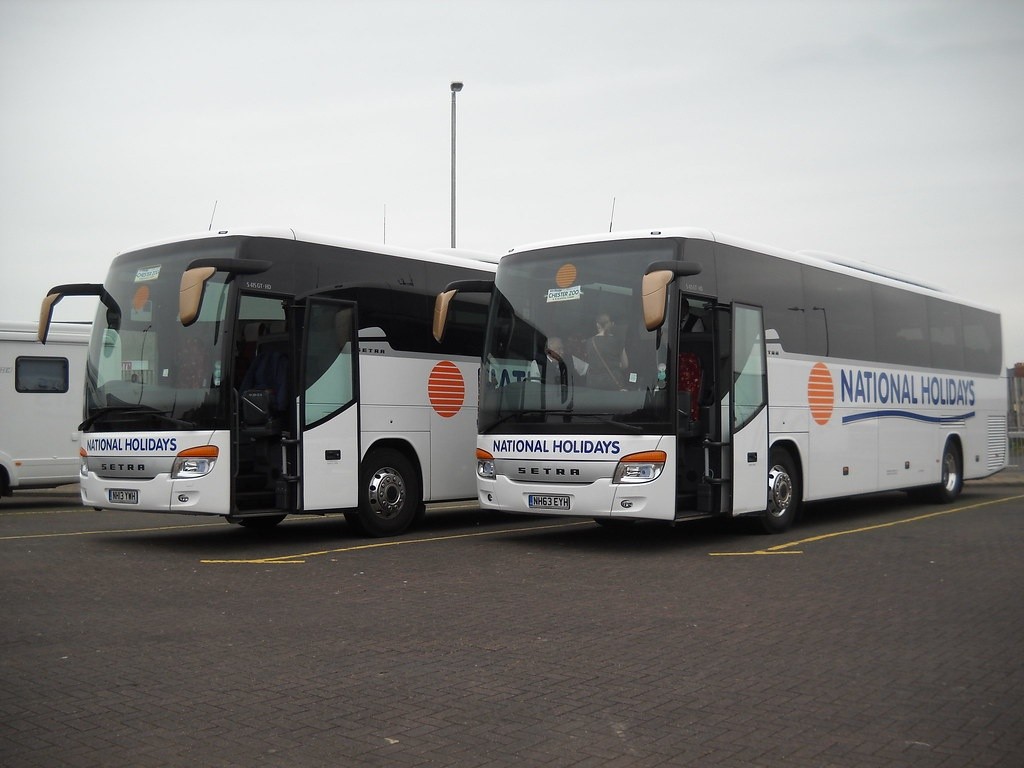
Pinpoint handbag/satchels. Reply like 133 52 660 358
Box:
617 381 632 391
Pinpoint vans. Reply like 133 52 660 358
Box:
0 320 92 495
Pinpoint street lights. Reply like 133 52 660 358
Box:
449 81 464 248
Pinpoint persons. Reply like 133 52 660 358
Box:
680 297 706 334
529 336 574 388
583 313 628 390
676 348 704 432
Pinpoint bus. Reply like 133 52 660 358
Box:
434 225 1010 534
38 228 501 540
